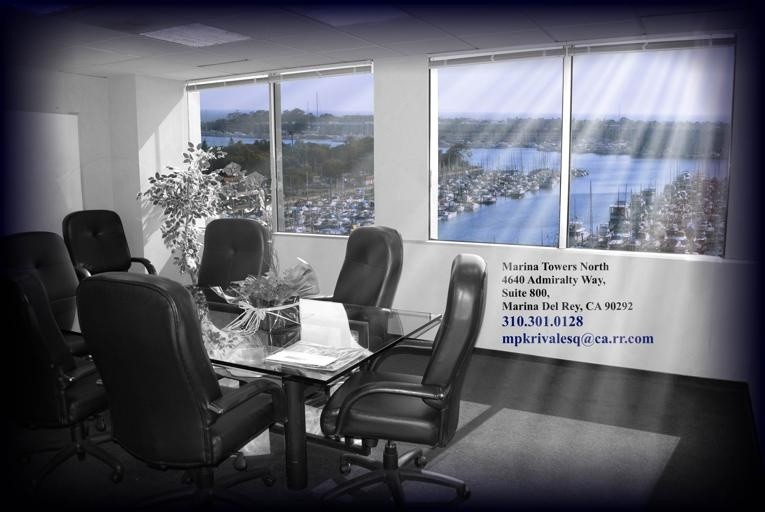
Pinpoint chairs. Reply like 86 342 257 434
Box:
0 209 489 512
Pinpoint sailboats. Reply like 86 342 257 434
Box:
556 156 729 257
